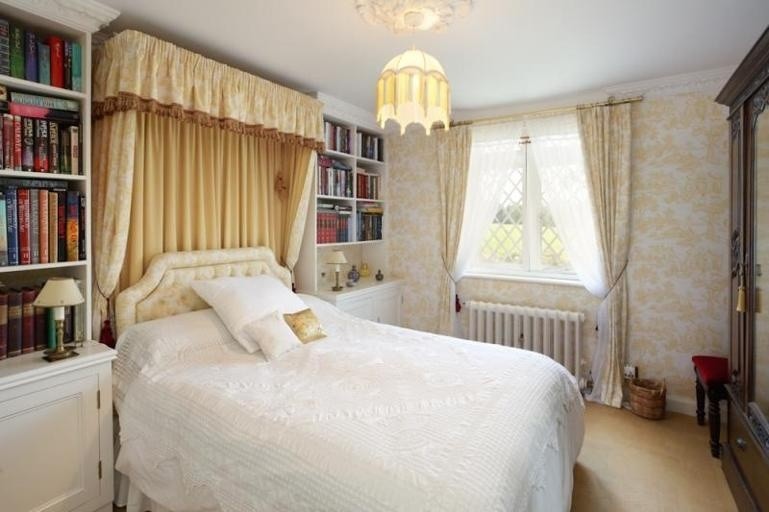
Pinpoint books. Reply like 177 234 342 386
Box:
1 11 87 360
317 118 383 246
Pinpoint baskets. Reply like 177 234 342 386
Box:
627 374 669 423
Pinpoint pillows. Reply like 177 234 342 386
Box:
191 273 327 362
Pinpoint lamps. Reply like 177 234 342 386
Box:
326 250 348 291
32 277 85 360
353 0 473 136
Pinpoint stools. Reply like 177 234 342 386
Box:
691 357 728 457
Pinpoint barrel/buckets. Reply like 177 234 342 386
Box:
630 379 667 419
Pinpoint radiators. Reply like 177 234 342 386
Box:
463 300 588 390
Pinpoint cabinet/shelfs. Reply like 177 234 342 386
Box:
295 104 401 326
0 0 121 512
715 25 769 512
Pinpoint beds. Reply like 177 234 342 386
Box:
113 246 586 512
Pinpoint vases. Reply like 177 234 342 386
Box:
359 262 369 278
375 269 383 281
348 264 360 282
